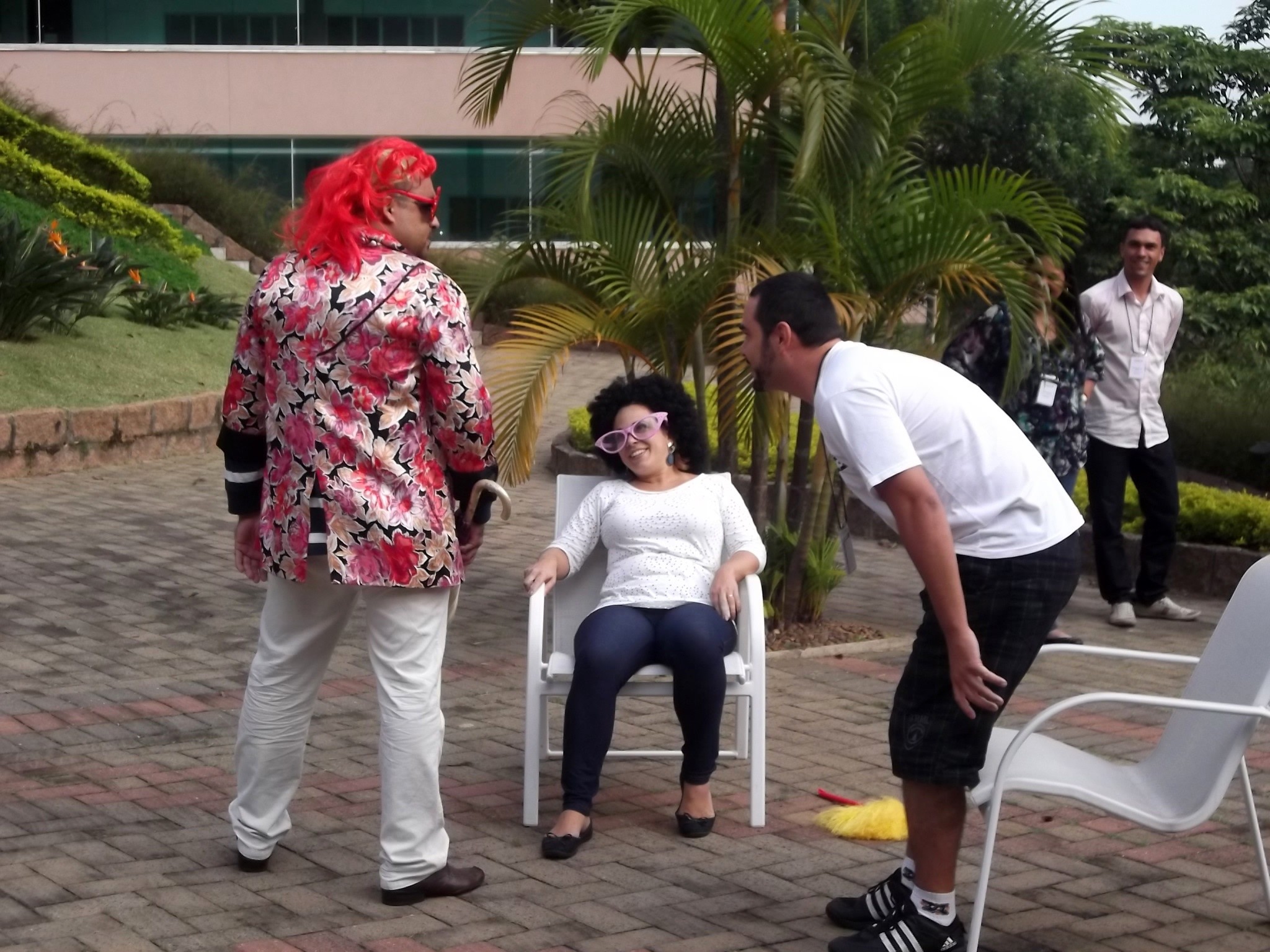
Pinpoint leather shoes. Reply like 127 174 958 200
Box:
238 849 267 872
380 864 485 905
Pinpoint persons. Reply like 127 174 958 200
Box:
942 216 1200 642
523 373 769 864
740 272 1086 952
218 138 486 907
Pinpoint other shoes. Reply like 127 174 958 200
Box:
1046 635 1085 643
543 817 593 860
1108 601 1136 626
675 778 716 837
1134 595 1201 620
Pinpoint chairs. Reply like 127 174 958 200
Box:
964 555 1270 952
522 471 767 832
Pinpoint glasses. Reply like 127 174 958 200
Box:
382 186 441 223
595 411 669 453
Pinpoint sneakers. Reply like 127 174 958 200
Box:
828 887 965 951
827 867 911 929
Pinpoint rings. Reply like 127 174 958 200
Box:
727 595 733 598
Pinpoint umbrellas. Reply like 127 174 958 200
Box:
447 479 512 625
